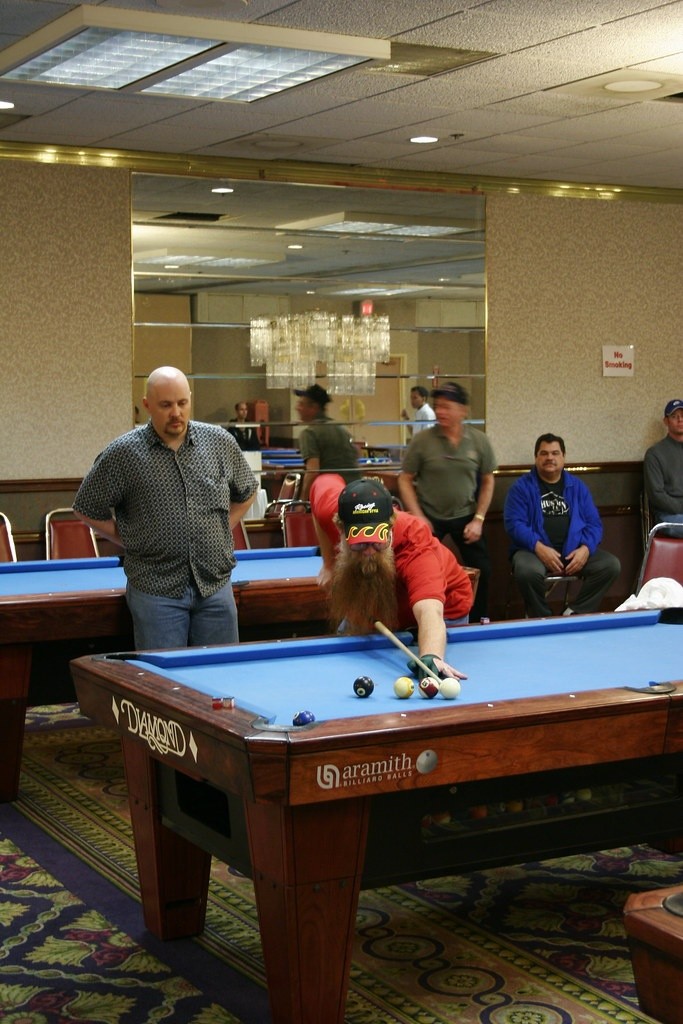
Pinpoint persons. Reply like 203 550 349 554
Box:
227 401 262 451
397 381 499 624
70 365 259 651
401 387 437 439
309 476 474 683
643 399 683 538
293 383 360 514
503 433 622 619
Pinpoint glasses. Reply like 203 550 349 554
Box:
349 540 391 553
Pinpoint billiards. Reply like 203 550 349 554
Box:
417 676 439 699
439 677 461 699
293 710 315 726
352 675 375 698
393 676 415 699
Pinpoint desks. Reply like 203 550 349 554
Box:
70 607 683 1024
0 546 480 801
261 447 391 468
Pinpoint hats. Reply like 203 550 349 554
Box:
664 399 683 417
339 480 395 544
292 384 332 405
432 381 472 405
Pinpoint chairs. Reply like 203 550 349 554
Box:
264 473 301 516
0 499 683 705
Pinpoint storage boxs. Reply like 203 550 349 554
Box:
135 292 193 424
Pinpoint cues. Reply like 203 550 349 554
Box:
372 619 443 684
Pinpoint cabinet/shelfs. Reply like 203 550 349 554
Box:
194 290 485 328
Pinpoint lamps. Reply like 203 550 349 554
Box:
250 307 390 396
276 211 487 237
134 248 287 269
0 4 392 106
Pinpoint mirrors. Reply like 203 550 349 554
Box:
126 170 489 531
